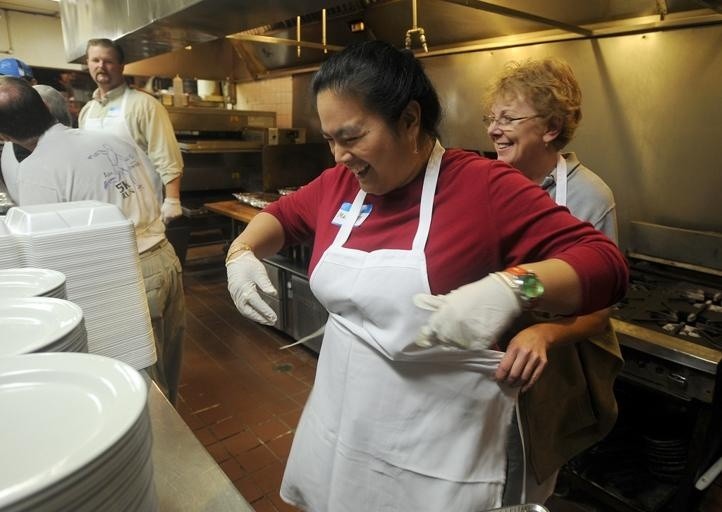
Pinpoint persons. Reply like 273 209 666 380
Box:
474 51 623 507
1 75 188 412
218 27 630 512
72 36 185 227
0 55 84 208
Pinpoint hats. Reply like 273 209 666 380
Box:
0 58 34 78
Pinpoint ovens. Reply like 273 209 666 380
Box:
556 362 720 510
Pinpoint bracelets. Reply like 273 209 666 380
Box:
223 239 252 261
494 271 529 314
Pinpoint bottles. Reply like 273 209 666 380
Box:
172 73 186 108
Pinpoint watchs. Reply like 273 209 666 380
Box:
503 263 545 315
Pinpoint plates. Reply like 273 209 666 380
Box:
0 267 158 508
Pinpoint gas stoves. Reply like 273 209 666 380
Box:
613 253 722 355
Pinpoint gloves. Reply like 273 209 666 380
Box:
413 273 521 350
160 196 182 225
225 251 278 326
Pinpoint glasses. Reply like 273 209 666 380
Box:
484 113 546 131
